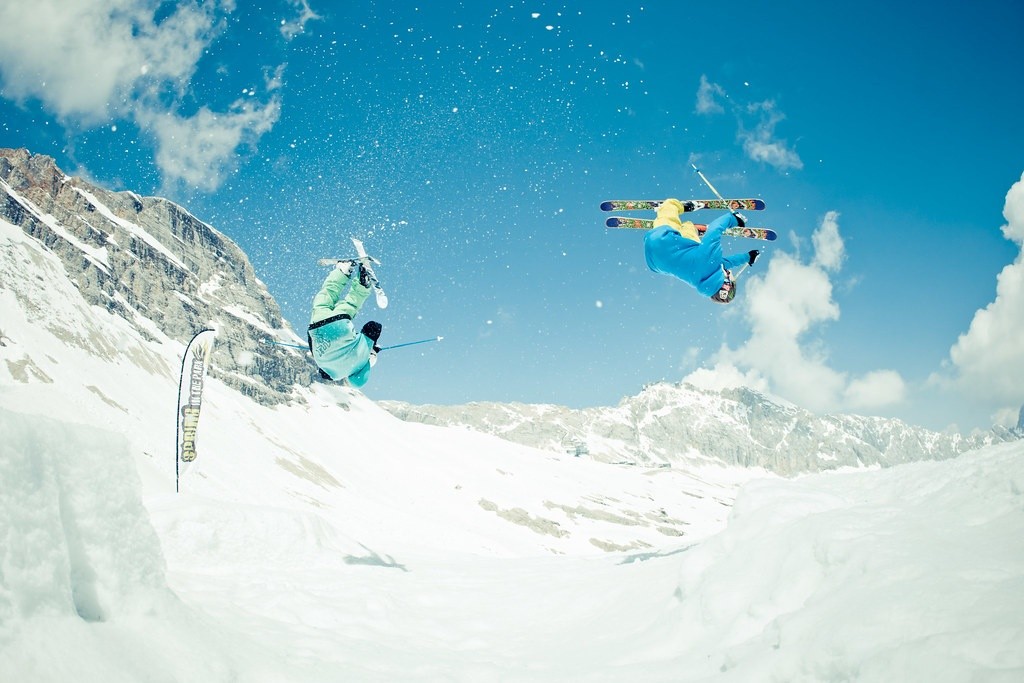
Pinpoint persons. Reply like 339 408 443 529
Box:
307 261 382 389
644 198 760 303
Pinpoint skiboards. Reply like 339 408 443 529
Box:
606 216 778 242
317 254 383 268
350 236 389 310
599 197 768 213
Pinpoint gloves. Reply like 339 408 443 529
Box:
749 248 762 264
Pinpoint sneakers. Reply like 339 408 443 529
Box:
681 200 703 215
336 261 356 270
358 264 367 287
